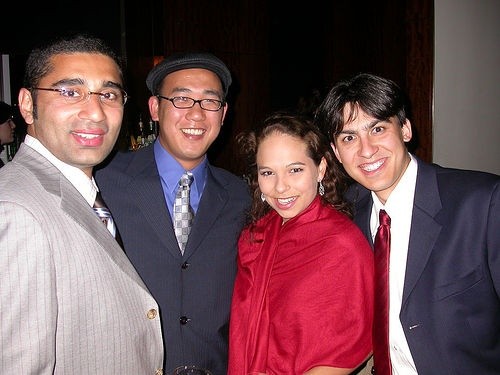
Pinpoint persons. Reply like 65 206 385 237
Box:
91 49 256 375
0 100 18 168
0 37 164 374
316 74 500 375
227 111 374 375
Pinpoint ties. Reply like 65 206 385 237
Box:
370 211 393 374
171 174 196 256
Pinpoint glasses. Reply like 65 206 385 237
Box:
31 84 129 108
157 92 225 112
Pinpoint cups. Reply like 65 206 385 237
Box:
171 366 213 375
